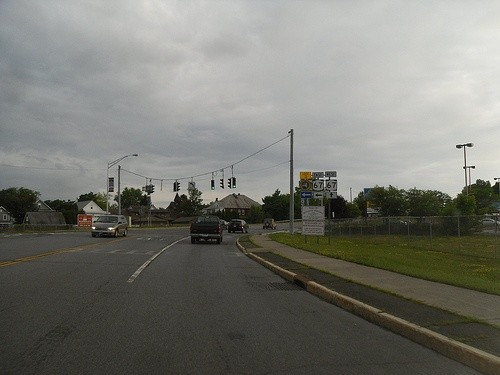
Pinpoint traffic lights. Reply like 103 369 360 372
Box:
233 177 237 188
220 179 225 189
228 178 232 189
151 185 155 193
176 181 181 192
210 179 215 190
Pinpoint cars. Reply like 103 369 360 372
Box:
228 218 249 234
91 214 127 239
220 220 231 230
479 218 500 228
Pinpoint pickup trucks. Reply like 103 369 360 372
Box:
190 215 225 244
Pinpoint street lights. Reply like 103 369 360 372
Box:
107 154 138 214
494 178 500 236
456 143 474 200
462 164 476 192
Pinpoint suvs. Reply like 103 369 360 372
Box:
263 218 278 230
